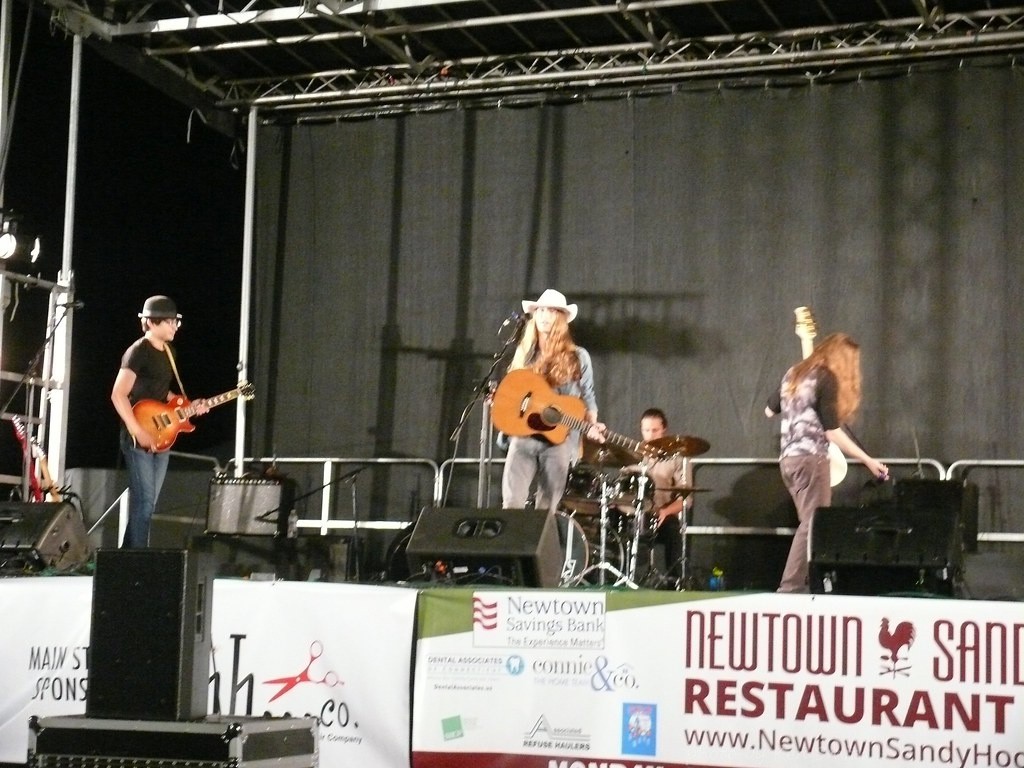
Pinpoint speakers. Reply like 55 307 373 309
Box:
809 504 968 600
205 477 299 536
406 505 565 588
893 477 980 556
85 549 215 722
0 500 95 575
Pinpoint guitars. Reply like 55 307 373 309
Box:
491 369 668 462
793 306 849 487
131 379 256 454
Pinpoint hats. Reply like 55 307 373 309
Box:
138 296 182 319
522 289 578 323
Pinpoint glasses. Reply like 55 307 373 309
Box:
163 318 182 327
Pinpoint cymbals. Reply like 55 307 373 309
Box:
581 435 635 466
646 435 711 457
655 485 713 492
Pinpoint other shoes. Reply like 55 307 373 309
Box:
776 584 816 595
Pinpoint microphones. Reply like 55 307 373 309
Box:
62 301 85 309
510 313 534 321
256 512 270 519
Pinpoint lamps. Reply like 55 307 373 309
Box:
0 208 42 263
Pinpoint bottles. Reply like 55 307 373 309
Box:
711 564 723 590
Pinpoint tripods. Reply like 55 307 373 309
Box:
563 453 705 591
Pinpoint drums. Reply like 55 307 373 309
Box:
554 510 624 588
562 465 609 515
614 472 656 515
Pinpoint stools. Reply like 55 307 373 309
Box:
631 531 682 590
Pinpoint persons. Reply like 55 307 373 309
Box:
499 290 604 517
622 407 697 589
110 296 210 551
768 332 888 593
